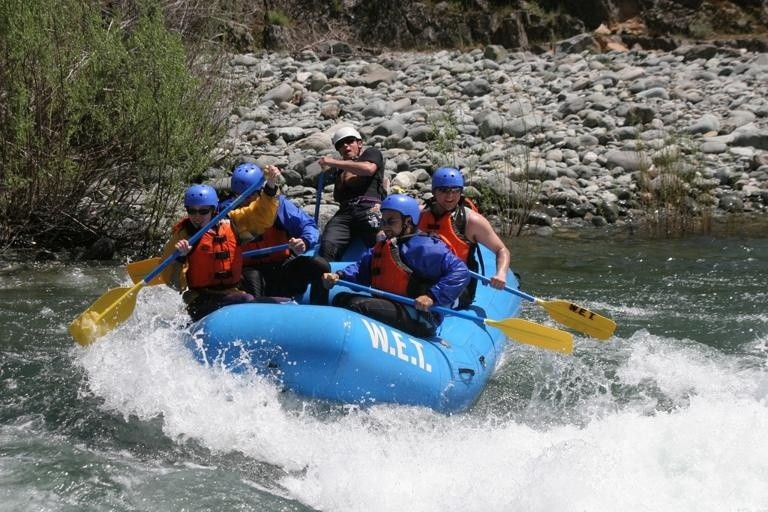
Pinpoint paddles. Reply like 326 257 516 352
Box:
126 242 293 287
68 171 265 345
332 275 574 355
467 266 617 341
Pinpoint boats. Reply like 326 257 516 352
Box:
182 244 524 416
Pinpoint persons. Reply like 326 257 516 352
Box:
375 166 512 311
160 162 291 323
172 162 332 306
320 191 472 340
318 126 389 264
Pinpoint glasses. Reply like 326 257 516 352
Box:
186 208 210 215
439 186 460 194
381 218 401 225
335 136 358 151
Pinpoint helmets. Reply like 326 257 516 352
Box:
432 165 464 195
231 162 264 192
333 126 362 144
184 184 219 214
380 192 420 225
